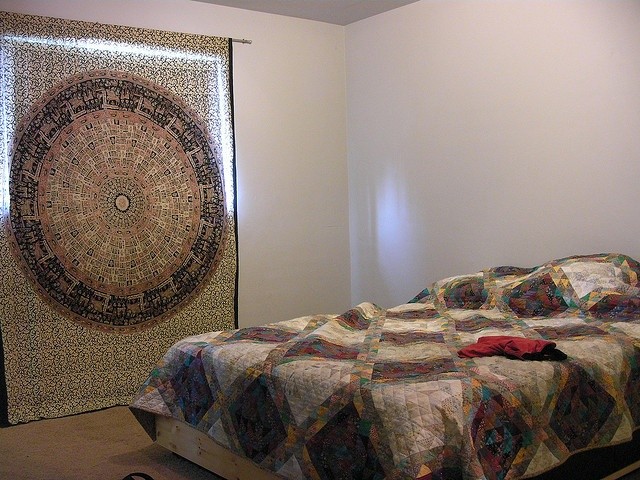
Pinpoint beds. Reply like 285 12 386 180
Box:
127 253 640 477
